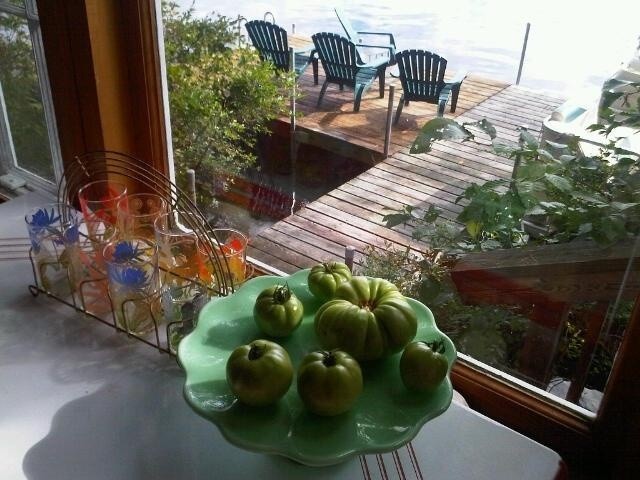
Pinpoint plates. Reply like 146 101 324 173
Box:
174 266 458 467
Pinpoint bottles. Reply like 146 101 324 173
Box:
116 192 169 246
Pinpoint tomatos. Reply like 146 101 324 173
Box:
399 338 448 392
296 349 364 418
314 278 417 357
308 261 352 299
253 281 304 338
226 339 294 409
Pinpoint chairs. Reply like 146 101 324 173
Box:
246 21 468 126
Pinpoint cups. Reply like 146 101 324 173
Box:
161 265 210 355
61 217 118 316
194 227 249 301
79 180 129 255
153 209 207 292
23 201 80 299
101 235 163 336
